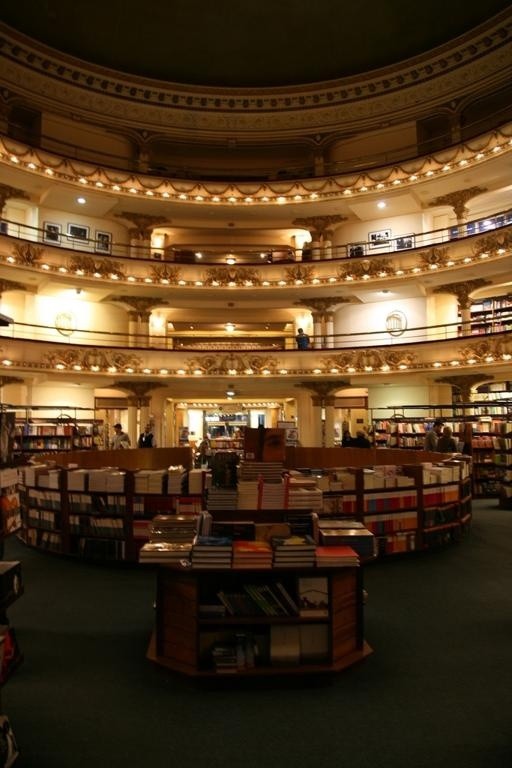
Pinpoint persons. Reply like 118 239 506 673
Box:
48 226 58 240
350 245 363 256
302 241 311 260
98 235 108 250
296 328 310 348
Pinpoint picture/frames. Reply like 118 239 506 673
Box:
346 240 366 258
393 232 415 252
43 220 63 246
67 222 90 244
94 230 112 256
367 228 392 251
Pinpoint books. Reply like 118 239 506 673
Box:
459 297 512 337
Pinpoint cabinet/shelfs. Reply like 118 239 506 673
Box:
16 454 471 571
135 544 376 691
0 406 105 464
364 403 511 497
